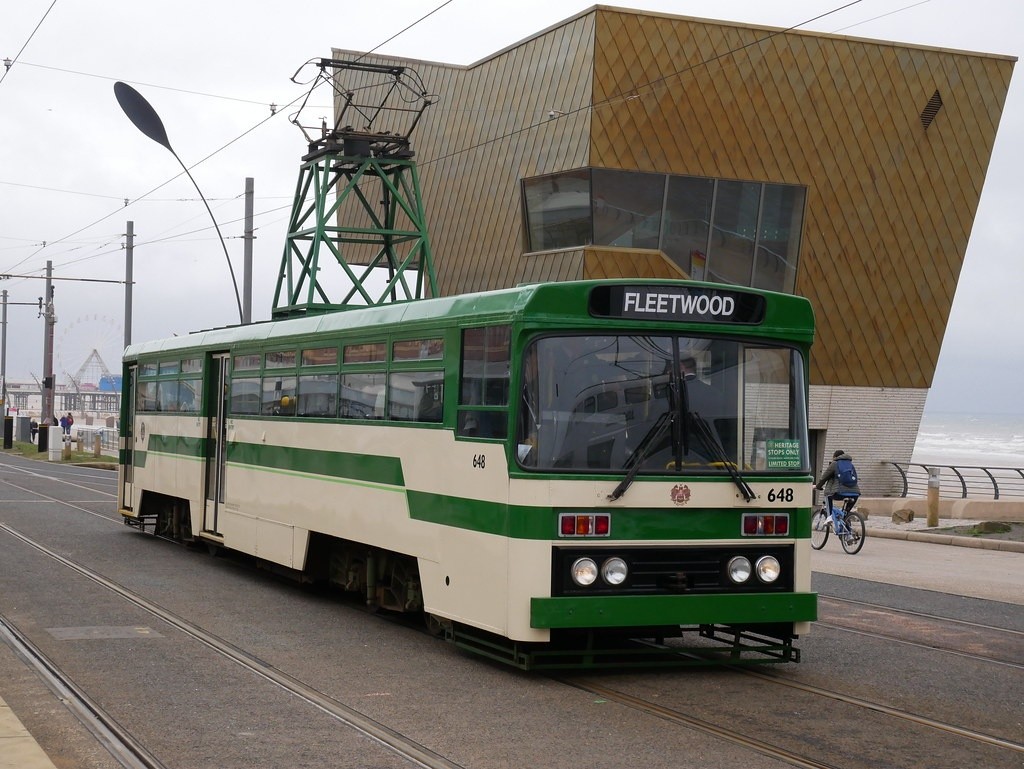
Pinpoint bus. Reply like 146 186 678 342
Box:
116 277 817 676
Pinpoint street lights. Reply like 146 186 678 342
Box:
113 82 242 322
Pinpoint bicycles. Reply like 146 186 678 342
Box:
811 486 866 554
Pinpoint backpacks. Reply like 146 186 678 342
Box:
67 416 74 425
835 459 858 488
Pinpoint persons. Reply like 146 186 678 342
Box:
567 376 627 432
66 412 73 434
812 450 860 543
30 418 39 442
60 415 66 434
675 354 723 465
54 415 58 426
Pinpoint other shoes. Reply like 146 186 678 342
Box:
822 515 833 527
